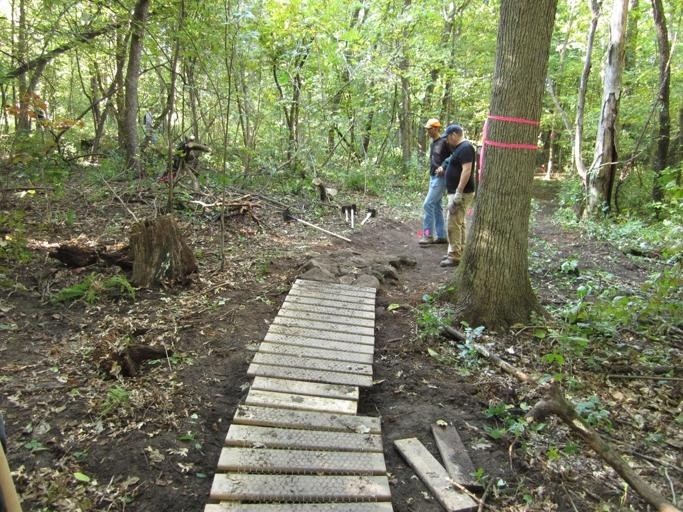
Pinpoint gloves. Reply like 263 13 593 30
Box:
453 192 463 204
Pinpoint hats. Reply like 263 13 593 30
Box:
440 126 462 138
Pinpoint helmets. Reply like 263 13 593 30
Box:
424 119 441 128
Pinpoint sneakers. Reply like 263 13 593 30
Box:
439 256 460 267
418 237 448 244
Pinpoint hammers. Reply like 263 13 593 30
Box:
283 210 351 243
342 205 375 228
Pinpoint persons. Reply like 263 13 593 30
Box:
418 119 475 267
141 107 158 144
44 98 49 119
174 135 211 172
